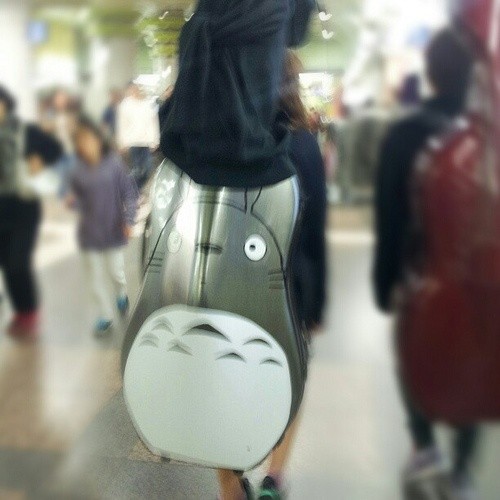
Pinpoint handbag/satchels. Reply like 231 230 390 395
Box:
15 123 60 198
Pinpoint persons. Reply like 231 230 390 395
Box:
216 50 326 500
64 120 136 335
28 88 74 202
104 82 160 186
367 26 487 500
0 83 63 336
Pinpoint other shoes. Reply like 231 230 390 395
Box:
95 320 112 335
402 446 448 481
258 476 282 500
7 310 37 337
117 296 130 316
240 479 252 500
434 469 469 499
